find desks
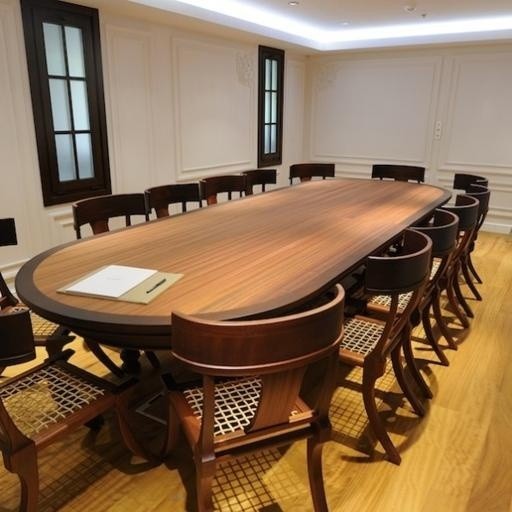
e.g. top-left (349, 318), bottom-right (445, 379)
top-left (14, 177), bottom-right (453, 449)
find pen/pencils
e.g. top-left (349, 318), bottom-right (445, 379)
top-left (147, 279), bottom-right (165, 293)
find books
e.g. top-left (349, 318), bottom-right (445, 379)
top-left (64, 265), bottom-right (158, 299)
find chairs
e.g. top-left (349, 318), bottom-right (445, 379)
top-left (73, 193), bottom-right (161, 368)
top-left (371, 163), bottom-right (427, 184)
top-left (3, 349), bottom-right (161, 510)
top-left (339, 228), bottom-right (425, 465)
top-left (3, 216), bottom-right (125, 380)
top-left (200, 176), bottom-right (252, 206)
top-left (290, 164), bottom-right (335, 190)
top-left (242, 169), bottom-right (277, 196)
top-left (142, 183), bottom-right (204, 223)
top-left (413, 174), bottom-right (490, 366)
top-left (161, 283), bottom-right (344, 512)
